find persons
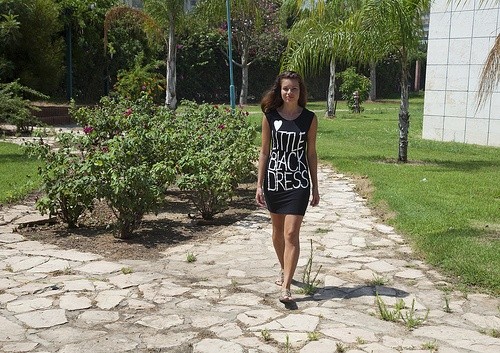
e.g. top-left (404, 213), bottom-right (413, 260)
top-left (255, 70), bottom-right (320, 304)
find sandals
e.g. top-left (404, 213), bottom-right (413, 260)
top-left (279, 288), bottom-right (293, 303)
top-left (276, 269), bottom-right (284, 286)
